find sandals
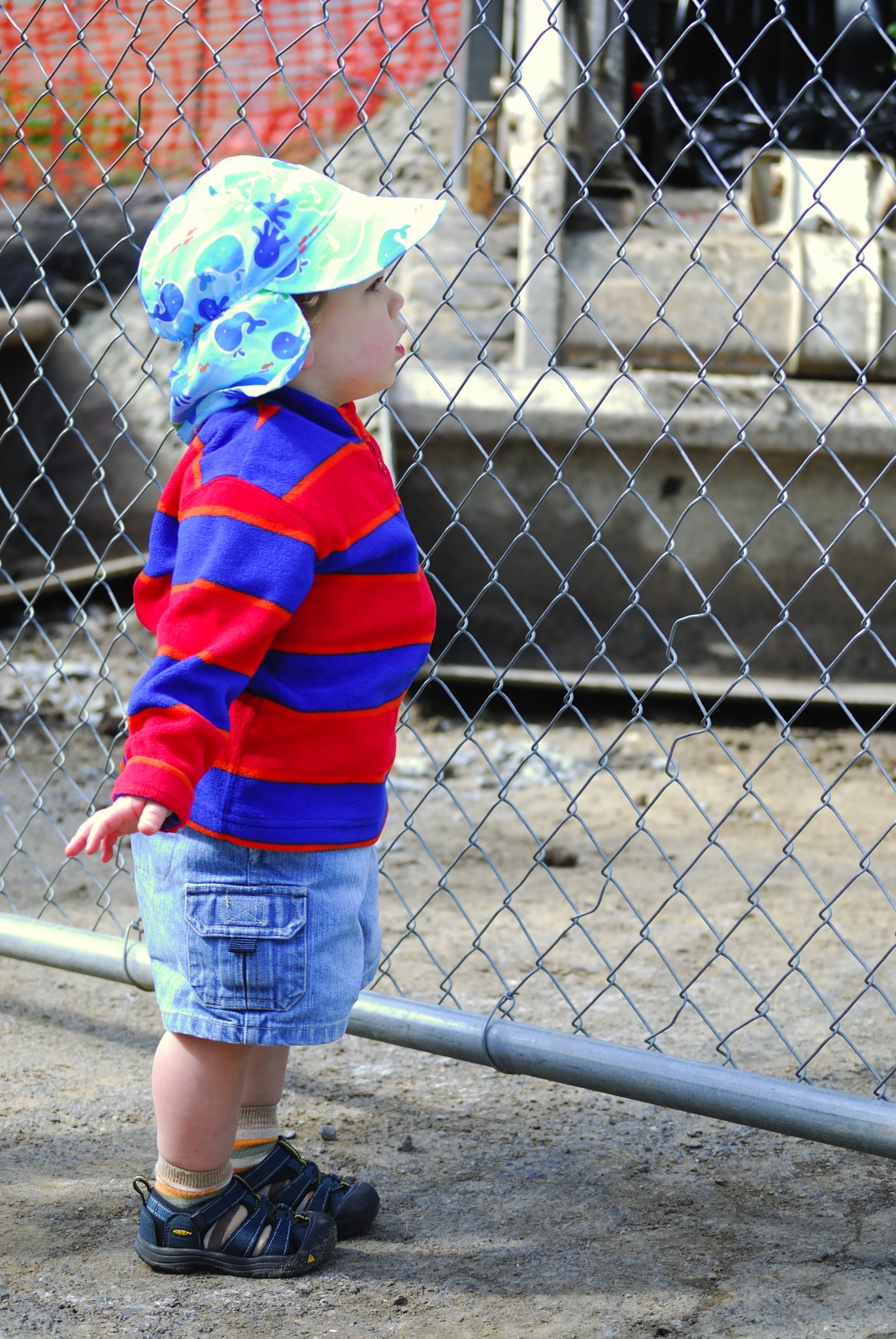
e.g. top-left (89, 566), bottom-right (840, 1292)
top-left (235, 1135), bottom-right (380, 1238)
top-left (132, 1172), bottom-right (337, 1279)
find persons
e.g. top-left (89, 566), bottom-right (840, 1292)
top-left (62, 156), bottom-right (438, 1278)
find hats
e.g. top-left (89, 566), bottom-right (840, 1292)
top-left (138, 155), bottom-right (447, 445)
